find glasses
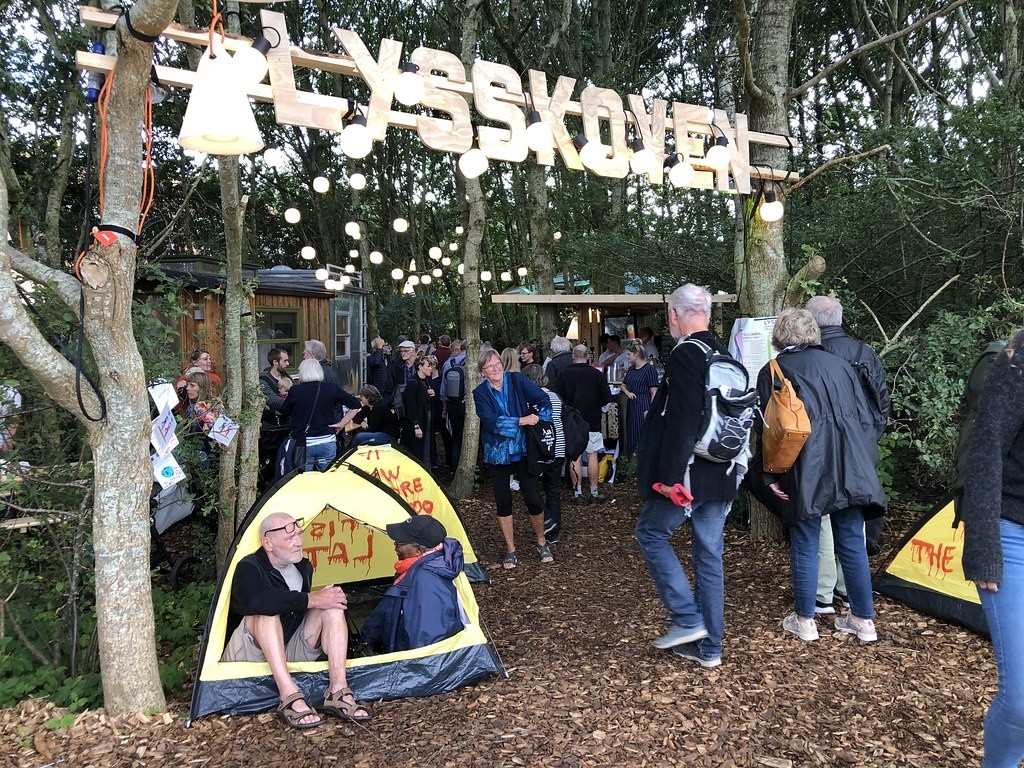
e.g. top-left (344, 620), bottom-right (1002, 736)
top-left (264, 518), bottom-right (305, 537)
top-left (481, 362), bottom-right (502, 370)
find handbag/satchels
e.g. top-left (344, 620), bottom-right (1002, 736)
top-left (560, 403), bottom-right (590, 491)
top-left (526, 404), bottom-right (556, 460)
top-left (316, 610), bottom-right (380, 662)
top-left (274, 425), bottom-right (310, 483)
top-left (762, 358), bottom-right (812, 473)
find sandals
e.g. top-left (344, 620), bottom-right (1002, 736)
top-left (322, 688), bottom-right (372, 720)
top-left (277, 693), bottom-right (324, 729)
top-left (502, 551), bottom-right (517, 569)
top-left (536, 542), bottom-right (554, 563)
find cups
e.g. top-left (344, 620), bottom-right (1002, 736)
top-left (627, 323), bottom-right (635, 339)
top-left (607, 362), bottom-right (618, 382)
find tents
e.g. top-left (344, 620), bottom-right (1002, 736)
top-left (323, 431), bottom-right (492, 586)
top-left (874, 493), bottom-right (992, 639)
top-left (187, 458), bottom-right (510, 729)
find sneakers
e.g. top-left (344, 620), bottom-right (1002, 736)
top-left (654, 625), bottom-right (709, 649)
top-left (782, 612), bottom-right (819, 641)
top-left (589, 494), bottom-right (605, 505)
top-left (834, 609), bottom-right (877, 641)
top-left (574, 494), bottom-right (589, 505)
top-left (672, 641), bottom-right (722, 667)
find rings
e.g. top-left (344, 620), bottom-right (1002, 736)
top-left (774, 492), bottom-right (775, 495)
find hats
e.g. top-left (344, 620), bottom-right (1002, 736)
top-left (397, 341), bottom-right (415, 349)
top-left (386, 515), bottom-right (447, 549)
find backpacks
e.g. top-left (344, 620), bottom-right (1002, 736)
top-left (670, 338), bottom-right (769, 463)
top-left (950, 337), bottom-right (1009, 528)
top-left (444, 357), bottom-right (465, 401)
top-left (826, 343), bottom-right (887, 442)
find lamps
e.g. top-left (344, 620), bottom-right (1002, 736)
top-left (178, 19), bottom-right (264, 155)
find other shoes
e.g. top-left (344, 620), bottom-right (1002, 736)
top-left (815, 599), bottom-right (835, 614)
top-left (833, 586), bottom-right (850, 608)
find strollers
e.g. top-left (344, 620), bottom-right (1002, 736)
top-left (257, 426), bottom-right (291, 493)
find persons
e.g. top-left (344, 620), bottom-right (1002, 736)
top-left (260, 326), bottom-right (663, 490)
top-left (959, 320), bottom-right (1024, 768)
top-left (221, 513), bottom-right (373, 728)
top-left (347, 514), bottom-right (463, 656)
top-left (473, 349), bottom-right (554, 570)
top-left (556, 343), bottom-right (612, 507)
top-left (1, 383), bottom-right (22, 451)
top-left (520, 364), bottom-right (567, 545)
top-left (804, 295), bottom-right (891, 615)
top-left (638, 282), bottom-right (739, 669)
top-left (171, 349), bottom-right (228, 462)
top-left (754, 306), bottom-right (879, 643)
